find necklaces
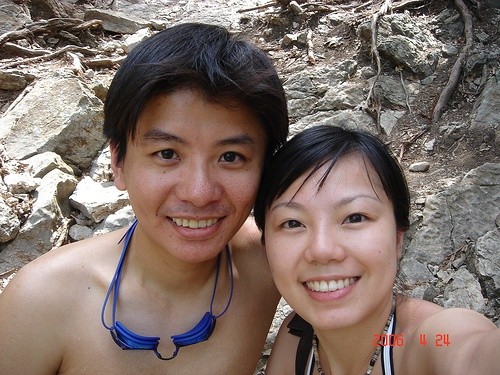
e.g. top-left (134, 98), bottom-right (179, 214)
top-left (311, 293), bottom-right (397, 375)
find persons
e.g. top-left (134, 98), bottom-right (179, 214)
top-left (255, 126), bottom-right (500, 375)
top-left (1, 24), bottom-right (290, 375)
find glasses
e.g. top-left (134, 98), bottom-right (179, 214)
top-left (110, 310), bottom-right (216, 360)
